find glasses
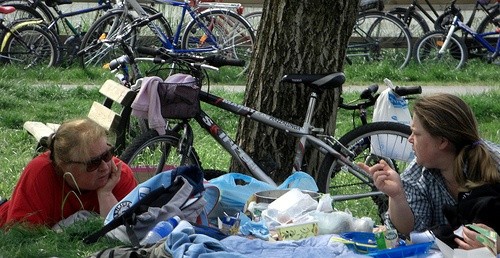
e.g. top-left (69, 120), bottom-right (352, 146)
top-left (68, 143), bottom-right (115, 172)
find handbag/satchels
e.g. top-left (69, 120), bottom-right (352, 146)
top-left (370, 78), bottom-right (417, 163)
top-left (103, 164), bottom-right (220, 245)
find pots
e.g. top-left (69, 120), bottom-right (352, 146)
top-left (254, 189), bottom-right (322, 204)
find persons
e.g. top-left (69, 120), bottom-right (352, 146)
top-left (0, 116), bottom-right (138, 234)
top-left (357, 92), bottom-right (500, 254)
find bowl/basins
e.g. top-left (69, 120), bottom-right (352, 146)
top-left (275, 220), bottom-right (319, 242)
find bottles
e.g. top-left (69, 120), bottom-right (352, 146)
top-left (383, 212), bottom-right (398, 249)
top-left (139, 215), bottom-right (182, 246)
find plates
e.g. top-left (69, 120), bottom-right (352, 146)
top-left (368, 240), bottom-right (435, 258)
top-left (340, 230), bottom-right (377, 253)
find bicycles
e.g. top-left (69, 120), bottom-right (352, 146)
top-left (1, 0), bottom-right (498, 227)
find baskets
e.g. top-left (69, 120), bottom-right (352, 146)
top-left (146, 68), bottom-right (201, 120)
